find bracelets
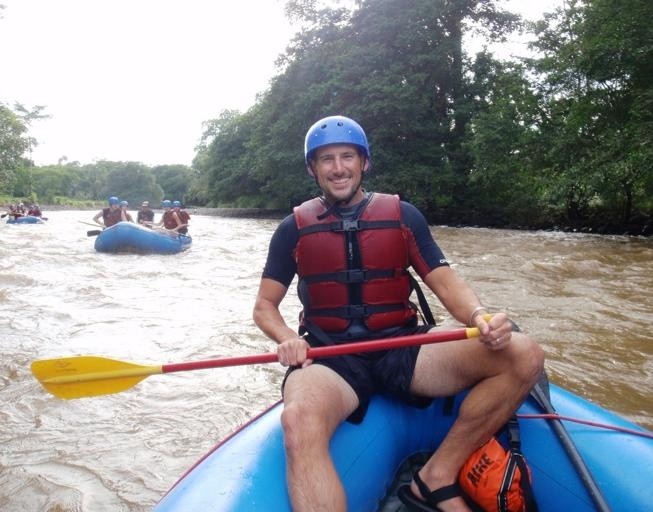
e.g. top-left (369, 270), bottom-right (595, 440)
top-left (467, 304), bottom-right (489, 326)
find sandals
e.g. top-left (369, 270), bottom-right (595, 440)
top-left (396, 469), bottom-right (466, 512)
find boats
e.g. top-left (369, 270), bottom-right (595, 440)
top-left (148, 376), bottom-right (653, 511)
top-left (5, 216), bottom-right (47, 224)
top-left (93, 221), bottom-right (192, 255)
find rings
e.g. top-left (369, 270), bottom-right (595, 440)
top-left (495, 337), bottom-right (501, 344)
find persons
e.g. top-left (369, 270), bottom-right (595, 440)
top-left (251, 113), bottom-right (547, 510)
top-left (8, 200), bottom-right (42, 219)
top-left (142, 200), bottom-right (182, 237)
top-left (136, 199), bottom-right (154, 230)
top-left (117, 200), bottom-right (135, 224)
top-left (91, 196), bottom-right (127, 229)
top-left (160, 201), bottom-right (191, 236)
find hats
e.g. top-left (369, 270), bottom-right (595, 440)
top-left (141, 201), bottom-right (149, 207)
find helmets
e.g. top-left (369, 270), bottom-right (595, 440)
top-left (108, 196), bottom-right (120, 207)
top-left (120, 201), bottom-right (129, 207)
top-left (9, 202), bottom-right (39, 212)
top-left (173, 201), bottom-right (182, 207)
top-left (161, 199), bottom-right (173, 208)
top-left (302, 112), bottom-right (371, 176)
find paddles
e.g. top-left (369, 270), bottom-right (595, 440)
top-left (87, 231), bottom-right (100, 236)
top-left (31, 314), bottom-right (492, 400)
top-left (1, 208), bottom-right (16, 218)
